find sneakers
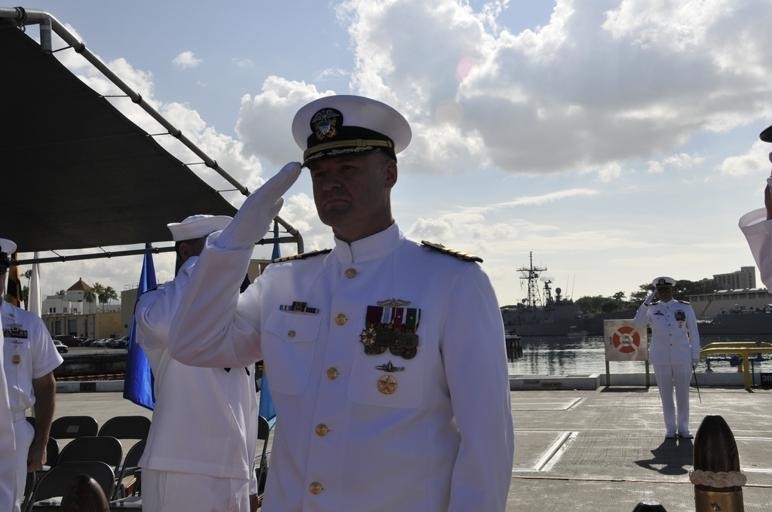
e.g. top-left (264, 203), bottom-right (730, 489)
top-left (664, 433), bottom-right (693, 441)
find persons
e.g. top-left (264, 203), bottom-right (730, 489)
top-left (633, 276), bottom-right (701, 439)
top-left (166, 95), bottom-right (515, 512)
top-left (133, 215), bottom-right (261, 512)
top-left (0, 238), bottom-right (57, 512)
top-left (739, 124), bottom-right (772, 293)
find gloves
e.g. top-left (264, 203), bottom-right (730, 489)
top-left (214, 161), bottom-right (302, 249)
top-left (644, 292), bottom-right (655, 306)
top-left (692, 359), bottom-right (701, 370)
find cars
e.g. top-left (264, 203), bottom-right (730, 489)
top-left (54, 335), bottom-right (129, 354)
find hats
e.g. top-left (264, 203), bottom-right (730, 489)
top-left (650, 277), bottom-right (678, 290)
top-left (0, 236), bottom-right (18, 274)
top-left (290, 94), bottom-right (414, 170)
top-left (167, 212), bottom-right (234, 243)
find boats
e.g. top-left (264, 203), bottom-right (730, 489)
top-left (696, 307), bottom-right (770, 337)
top-left (501, 247), bottom-right (580, 332)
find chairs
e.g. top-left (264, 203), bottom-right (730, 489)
top-left (21, 409), bottom-right (273, 512)
